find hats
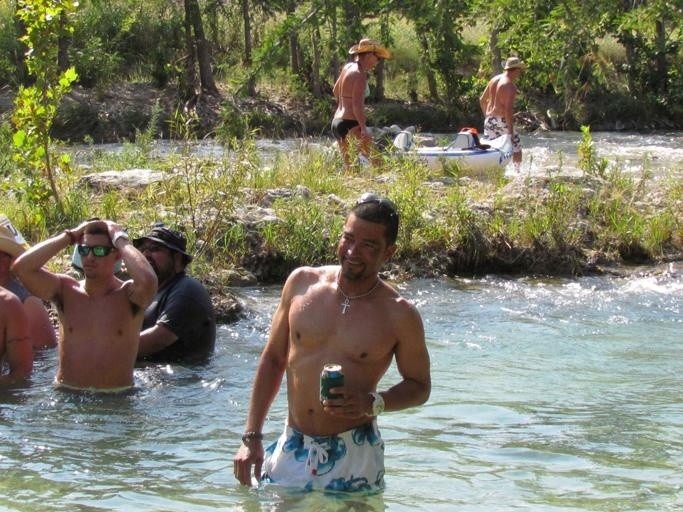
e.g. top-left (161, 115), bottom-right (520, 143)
top-left (500, 56), bottom-right (528, 70)
top-left (132, 226), bottom-right (192, 267)
top-left (348, 39), bottom-right (391, 59)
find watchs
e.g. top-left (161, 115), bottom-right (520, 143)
top-left (364, 390), bottom-right (384, 418)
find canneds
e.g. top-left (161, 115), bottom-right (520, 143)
top-left (319, 363), bottom-right (345, 408)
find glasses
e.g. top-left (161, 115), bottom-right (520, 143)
top-left (76, 244), bottom-right (118, 258)
top-left (355, 191), bottom-right (399, 218)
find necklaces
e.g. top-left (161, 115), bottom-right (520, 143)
top-left (336, 269), bottom-right (381, 315)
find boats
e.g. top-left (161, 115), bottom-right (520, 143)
top-left (364, 133), bottom-right (512, 175)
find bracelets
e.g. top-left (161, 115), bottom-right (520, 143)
top-left (240, 431), bottom-right (266, 442)
top-left (64, 228), bottom-right (75, 247)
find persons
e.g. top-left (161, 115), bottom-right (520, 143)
top-left (0, 286), bottom-right (34, 386)
top-left (12, 216), bottom-right (159, 392)
top-left (118, 225), bottom-right (216, 362)
top-left (330, 38), bottom-right (392, 172)
top-left (478, 56), bottom-right (527, 175)
top-left (0, 214), bottom-right (58, 349)
top-left (232, 199), bottom-right (432, 495)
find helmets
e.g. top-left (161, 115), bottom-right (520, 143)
top-left (0, 213), bottom-right (30, 259)
